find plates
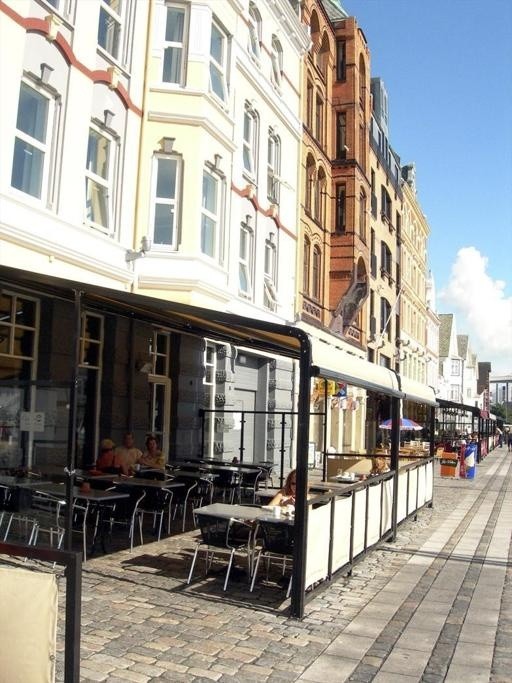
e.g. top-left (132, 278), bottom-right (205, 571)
top-left (262, 506), bottom-right (285, 510)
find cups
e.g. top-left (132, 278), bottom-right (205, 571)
top-left (273, 506), bottom-right (279, 516)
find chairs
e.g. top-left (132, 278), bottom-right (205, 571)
top-left (144, 486), bottom-right (174, 542)
top-left (0, 482), bottom-right (16, 542)
top-left (249, 519), bottom-right (296, 599)
top-left (91, 484), bottom-right (145, 552)
top-left (212, 468), bottom-right (243, 505)
top-left (175, 480), bottom-right (198, 533)
top-left (244, 471), bottom-right (262, 504)
top-left (14, 485), bottom-right (67, 550)
top-left (187, 512), bottom-right (261, 592)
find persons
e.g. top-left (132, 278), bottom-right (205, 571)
top-left (95, 437), bottom-right (124, 475)
top-left (267, 469), bottom-right (296, 509)
top-left (507, 432), bottom-right (512, 452)
top-left (499, 432), bottom-right (503, 448)
top-left (115, 432), bottom-right (140, 475)
top-left (139, 435), bottom-right (166, 471)
top-left (367, 453), bottom-right (390, 481)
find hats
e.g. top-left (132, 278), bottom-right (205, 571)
top-left (101, 440), bottom-right (116, 449)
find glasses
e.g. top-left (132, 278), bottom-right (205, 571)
top-left (290, 482), bottom-right (297, 485)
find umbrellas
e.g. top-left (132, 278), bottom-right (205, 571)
top-left (379, 417), bottom-right (423, 432)
